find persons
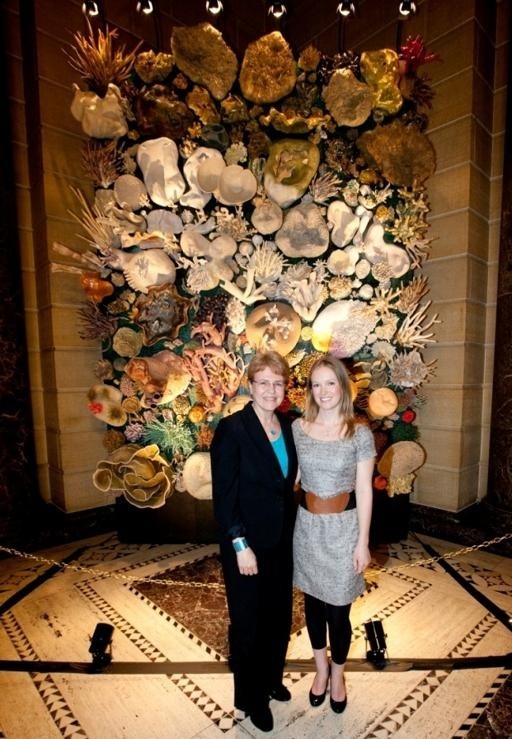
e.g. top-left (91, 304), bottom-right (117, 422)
top-left (207, 350), bottom-right (307, 733)
top-left (289, 355), bottom-right (379, 714)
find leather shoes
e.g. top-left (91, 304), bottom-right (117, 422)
top-left (330, 693), bottom-right (346, 714)
top-left (248, 702), bottom-right (273, 731)
top-left (268, 683), bottom-right (291, 701)
top-left (309, 688), bottom-right (326, 706)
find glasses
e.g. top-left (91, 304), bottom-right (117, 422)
top-left (251, 379), bottom-right (285, 388)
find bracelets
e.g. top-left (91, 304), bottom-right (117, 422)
top-left (232, 540), bottom-right (252, 556)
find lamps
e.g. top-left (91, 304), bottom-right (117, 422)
top-left (364, 620), bottom-right (389, 671)
top-left (87, 622), bottom-right (115, 672)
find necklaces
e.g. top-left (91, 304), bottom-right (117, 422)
top-left (252, 403), bottom-right (277, 438)
